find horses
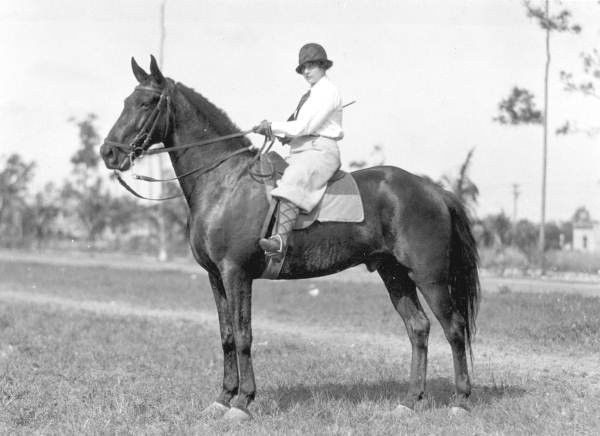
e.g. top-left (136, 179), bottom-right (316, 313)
top-left (99, 53), bottom-right (481, 423)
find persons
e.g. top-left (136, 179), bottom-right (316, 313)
top-left (253, 42), bottom-right (353, 263)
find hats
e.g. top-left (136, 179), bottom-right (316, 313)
top-left (295, 43), bottom-right (333, 73)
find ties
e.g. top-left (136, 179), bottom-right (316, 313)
top-left (289, 89), bottom-right (311, 120)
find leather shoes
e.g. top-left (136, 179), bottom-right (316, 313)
top-left (258, 239), bottom-right (287, 263)
